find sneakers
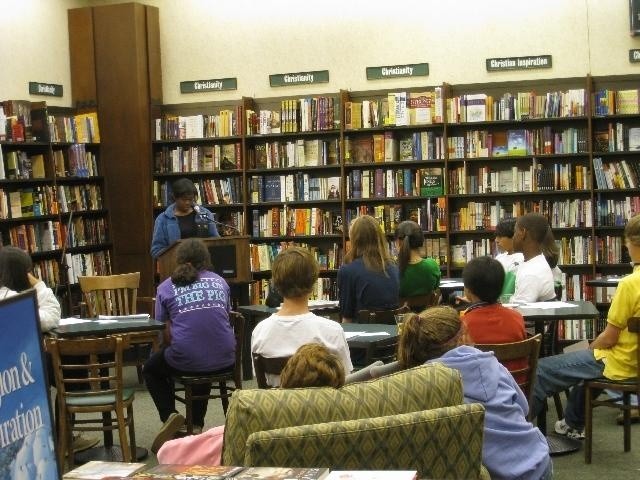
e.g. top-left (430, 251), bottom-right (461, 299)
top-left (65, 431), bottom-right (101, 456)
top-left (616, 409), bottom-right (640, 425)
top-left (555, 417), bottom-right (586, 440)
top-left (177, 422), bottom-right (202, 435)
top-left (151, 413), bottom-right (185, 454)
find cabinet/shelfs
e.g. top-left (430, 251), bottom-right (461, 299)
top-left (0, 97), bottom-right (120, 318)
top-left (146, 73), bottom-right (640, 354)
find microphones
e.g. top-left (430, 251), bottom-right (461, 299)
top-left (194, 205), bottom-right (207, 221)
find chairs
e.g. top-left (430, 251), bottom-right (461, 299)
top-left (45, 334), bottom-right (136, 474)
top-left (167, 311), bottom-right (246, 436)
top-left (77, 272), bottom-right (151, 384)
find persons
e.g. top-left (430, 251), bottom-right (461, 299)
top-left (457, 257), bottom-right (531, 405)
top-left (157, 343), bottom-right (346, 465)
top-left (1, 245), bottom-right (100, 457)
top-left (604, 387), bottom-right (640, 424)
top-left (398, 308), bottom-right (554, 480)
top-left (524, 217), bottom-right (640, 441)
top-left (250, 248), bottom-right (354, 389)
top-left (142, 239), bottom-right (236, 454)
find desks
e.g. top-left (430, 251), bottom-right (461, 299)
top-left (42, 315), bottom-right (167, 465)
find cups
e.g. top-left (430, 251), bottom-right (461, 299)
top-left (500, 293), bottom-right (513, 311)
top-left (394, 313), bottom-right (406, 335)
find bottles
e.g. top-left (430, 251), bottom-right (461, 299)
top-left (79, 302), bottom-right (86, 319)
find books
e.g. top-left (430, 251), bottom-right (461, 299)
top-left (64, 461), bottom-right (329, 480)
top-left (0, 88), bottom-right (640, 338)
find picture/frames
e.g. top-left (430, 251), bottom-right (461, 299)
top-left (0, 289), bottom-right (61, 480)
